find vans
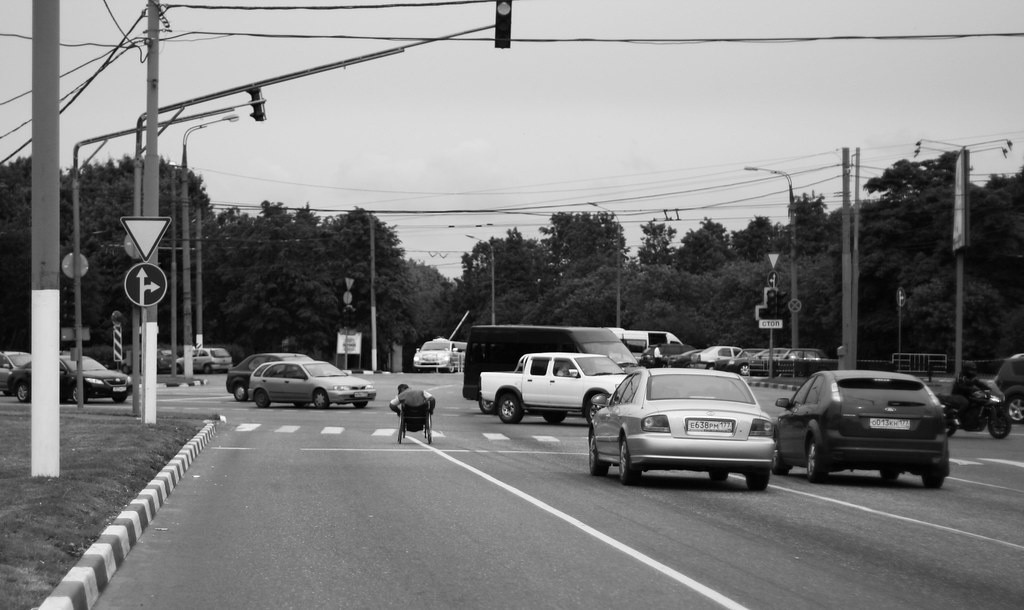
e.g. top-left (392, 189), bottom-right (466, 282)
top-left (612, 327), bottom-right (681, 364)
top-left (461, 325), bottom-right (639, 412)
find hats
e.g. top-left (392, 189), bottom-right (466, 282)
top-left (397, 384), bottom-right (408, 394)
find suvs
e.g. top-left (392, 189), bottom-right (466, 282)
top-left (412, 340), bottom-right (467, 373)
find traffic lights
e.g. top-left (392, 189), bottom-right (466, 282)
top-left (495, 1), bottom-right (513, 49)
top-left (247, 89), bottom-right (264, 121)
top-left (761, 290), bottom-right (790, 321)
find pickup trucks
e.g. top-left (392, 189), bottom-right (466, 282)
top-left (479, 352), bottom-right (631, 425)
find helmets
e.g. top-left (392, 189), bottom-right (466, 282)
top-left (962, 362), bottom-right (977, 377)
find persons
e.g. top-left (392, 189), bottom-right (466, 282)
top-left (556, 362), bottom-right (570, 377)
top-left (390, 384), bottom-right (436, 417)
top-left (654, 344), bottom-right (662, 367)
top-left (951, 361), bottom-right (993, 426)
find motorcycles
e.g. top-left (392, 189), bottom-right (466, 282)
top-left (939, 376), bottom-right (1011, 440)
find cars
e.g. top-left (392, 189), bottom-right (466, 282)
top-left (640, 344), bottom-right (830, 375)
top-left (586, 366), bottom-right (774, 490)
top-left (995, 355), bottom-right (1024, 421)
top-left (0, 350), bottom-right (31, 393)
top-left (7, 357), bottom-right (133, 405)
top-left (772, 374), bottom-right (951, 490)
top-left (226, 352), bottom-right (378, 408)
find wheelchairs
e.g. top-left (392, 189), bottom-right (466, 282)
top-left (398, 401), bottom-right (432, 444)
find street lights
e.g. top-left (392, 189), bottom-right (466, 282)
top-left (181, 115), bottom-right (240, 379)
top-left (745, 168), bottom-right (799, 348)
top-left (465, 235), bottom-right (495, 326)
top-left (588, 203), bottom-right (620, 327)
